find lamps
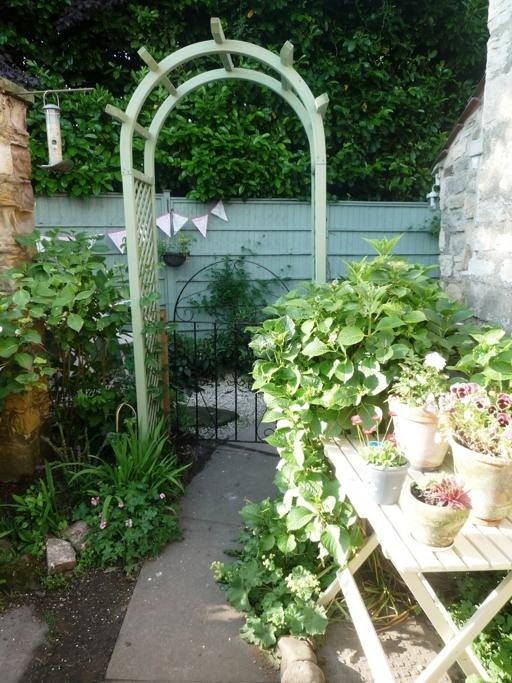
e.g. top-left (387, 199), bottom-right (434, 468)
top-left (425, 183), bottom-right (439, 210)
top-left (158, 231), bottom-right (191, 267)
top-left (43, 88), bottom-right (63, 166)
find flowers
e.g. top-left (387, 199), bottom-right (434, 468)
top-left (351, 407), bottom-right (409, 466)
top-left (438, 382), bottom-right (512, 460)
top-left (423, 472), bottom-right (472, 510)
top-left (385, 349), bottom-right (449, 407)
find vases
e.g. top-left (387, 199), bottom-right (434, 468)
top-left (447, 434), bottom-right (511, 523)
top-left (388, 398), bottom-right (451, 470)
top-left (409, 482), bottom-right (473, 551)
top-left (360, 456), bottom-right (411, 506)
